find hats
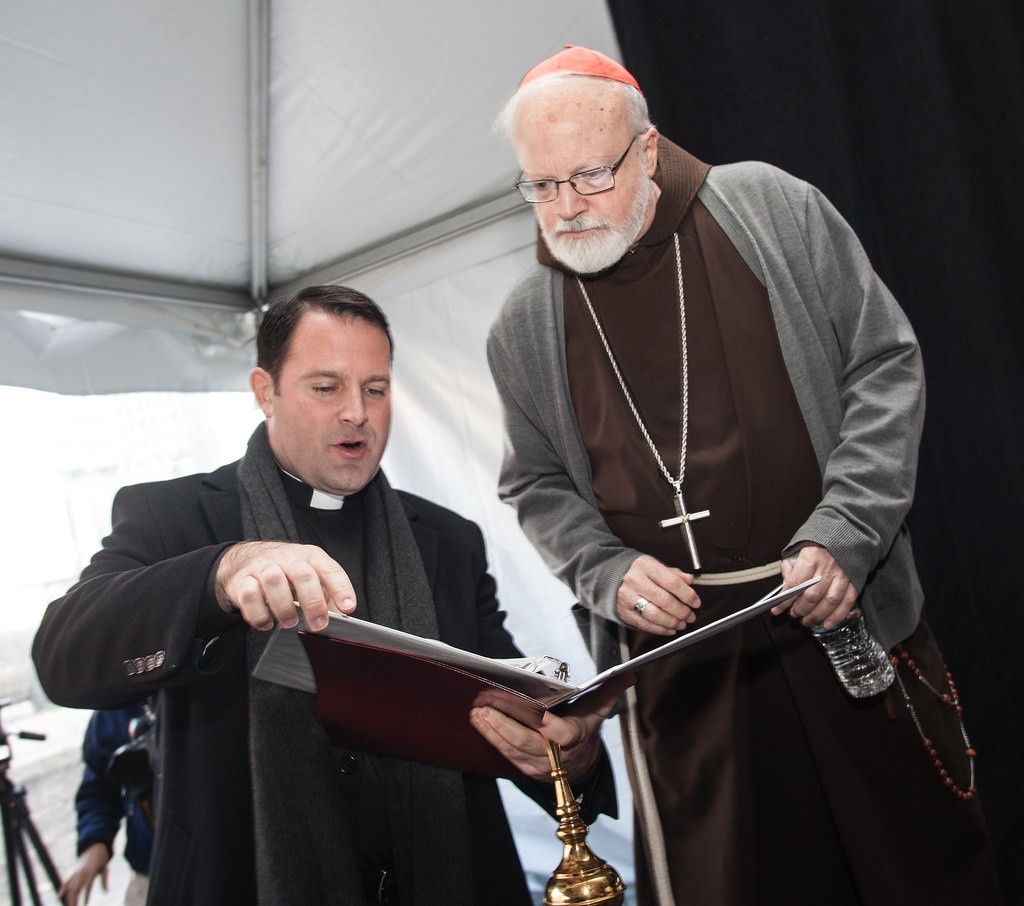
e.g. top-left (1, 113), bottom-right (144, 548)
top-left (517, 44), bottom-right (641, 90)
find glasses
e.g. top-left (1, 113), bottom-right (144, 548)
top-left (513, 131), bottom-right (637, 204)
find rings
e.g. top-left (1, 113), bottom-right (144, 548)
top-left (634, 598), bottom-right (650, 614)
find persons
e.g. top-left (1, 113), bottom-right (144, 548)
top-left (480, 46), bottom-right (1002, 906)
top-left (56, 702), bottom-right (161, 906)
top-left (34, 280), bottom-right (624, 906)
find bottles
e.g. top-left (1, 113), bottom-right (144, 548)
top-left (789, 554), bottom-right (896, 699)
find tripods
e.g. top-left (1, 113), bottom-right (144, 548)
top-left (0, 732), bottom-right (71, 906)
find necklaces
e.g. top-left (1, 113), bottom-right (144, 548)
top-left (577, 232), bottom-right (711, 571)
